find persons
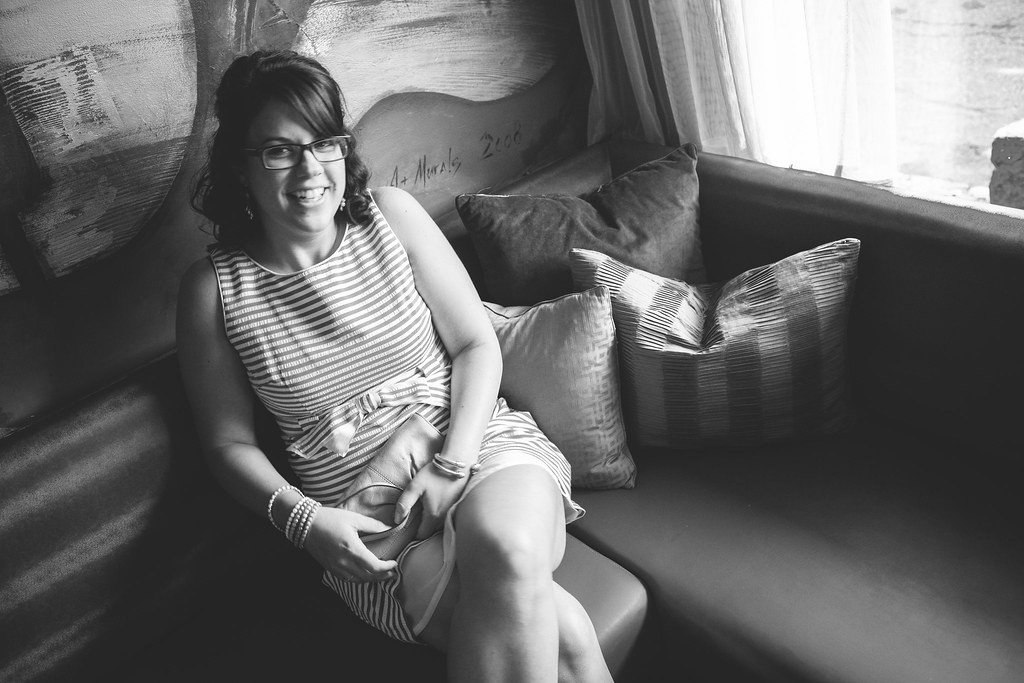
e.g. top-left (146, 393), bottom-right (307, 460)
top-left (176, 49), bottom-right (614, 683)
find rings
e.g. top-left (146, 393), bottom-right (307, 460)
top-left (344, 575), bottom-right (354, 584)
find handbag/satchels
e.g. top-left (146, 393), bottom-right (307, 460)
top-left (333, 412), bottom-right (444, 561)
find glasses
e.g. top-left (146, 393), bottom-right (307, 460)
top-left (242, 135), bottom-right (351, 169)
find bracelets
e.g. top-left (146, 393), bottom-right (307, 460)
top-left (267, 485), bottom-right (305, 532)
top-left (432, 452), bottom-right (483, 479)
top-left (285, 495), bottom-right (323, 550)
